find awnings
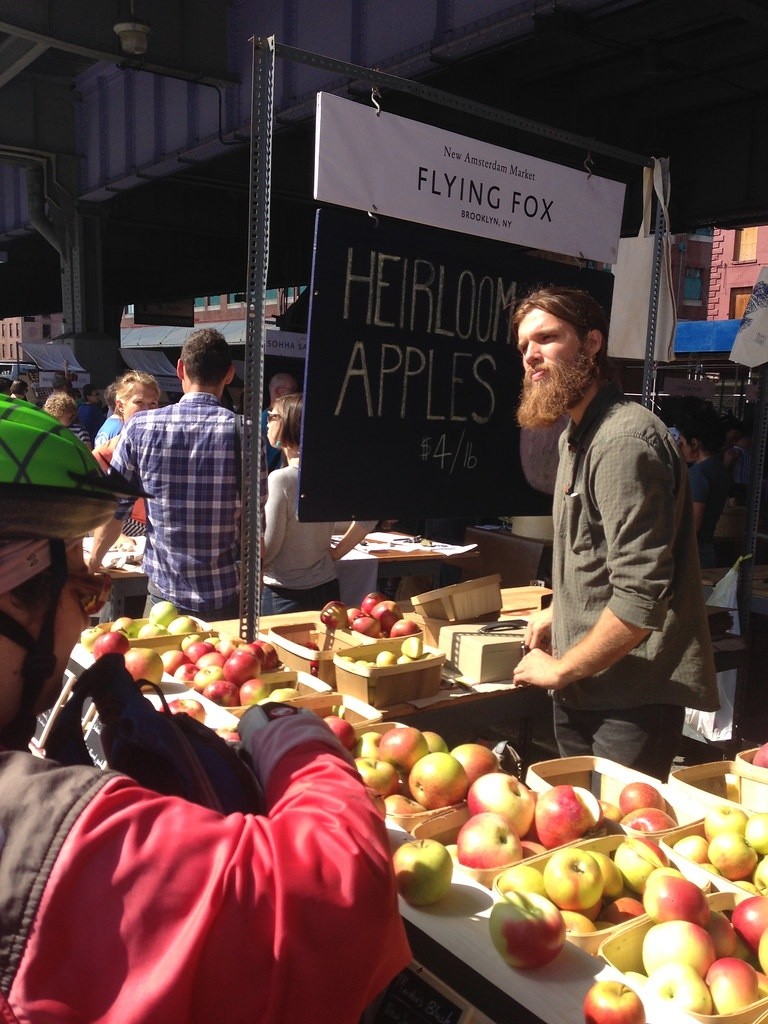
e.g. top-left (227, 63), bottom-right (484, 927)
top-left (15, 342), bottom-right (86, 382)
top-left (117, 348), bottom-right (179, 378)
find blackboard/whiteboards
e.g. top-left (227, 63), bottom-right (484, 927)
top-left (295, 206), bottom-right (621, 528)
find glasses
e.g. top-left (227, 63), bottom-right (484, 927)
top-left (41, 569), bottom-right (114, 615)
top-left (266, 410), bottom-right (281, 423)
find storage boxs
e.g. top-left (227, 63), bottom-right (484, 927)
top-left (189, 668), bottom-right (767, 1023)
top-left (268, 618), bottom-right (447, 710)
top-left (95, 613), bottom-right (219, 648)
top-left (410, 571), bottom-right (503, 649)
top-left (439, 620), bottom-right (530, 684)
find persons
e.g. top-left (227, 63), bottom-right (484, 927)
top-left (0, 391), bottom-right (412, 1024)
top-left (661, 397), bottom-right (755, 571)
top-left (0, 330), bottom-right (400, 622)
top-left (511, 285), bottom-right (723, 784)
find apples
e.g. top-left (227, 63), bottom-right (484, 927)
top-left (323, 714), bottom-right (768, 1024)
top-left (81, 590), bottom-right (436, 743)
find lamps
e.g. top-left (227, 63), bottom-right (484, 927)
top-left (112, 0), bottom-right (152, 55)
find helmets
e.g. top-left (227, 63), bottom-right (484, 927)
top-left (0, 393), bottom-right (155, 543)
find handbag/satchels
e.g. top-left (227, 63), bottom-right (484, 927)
top-left (43, 652), bottom-right (265, 820)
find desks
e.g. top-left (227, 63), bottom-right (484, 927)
top-left (81, 529), bottom-right (481, 624)
top-left (700, 565), bottom-right (768, 617)
top-left (384, 813), bottom-right (698, 1024)
top-left (78, 583), bottom-right (754, 783)
